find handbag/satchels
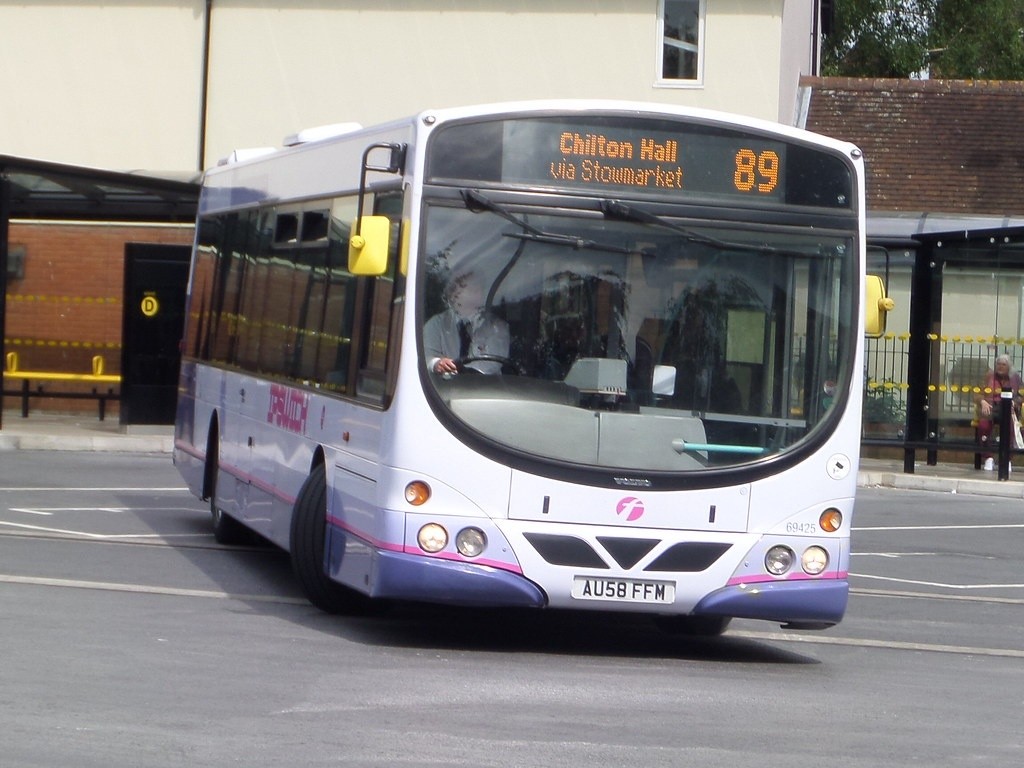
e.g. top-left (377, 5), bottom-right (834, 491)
top-left (1010, 411), bottom-right (1024, 449)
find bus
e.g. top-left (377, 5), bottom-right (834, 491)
top-left (171, 97), bottom-right (896, 638)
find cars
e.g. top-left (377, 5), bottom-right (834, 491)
top-left (948, 355), bottom-right (991, 401)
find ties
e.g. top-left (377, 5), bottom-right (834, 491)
top-left (458, 319), bottom-right (473, 359)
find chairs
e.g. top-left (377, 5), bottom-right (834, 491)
top-left (586, 332), bottom-right (657, 408)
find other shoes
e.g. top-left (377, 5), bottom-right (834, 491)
top-left (1009, 462), bottom-right (1012, 472)
top-left (984, 457), bottom-right (995, 471)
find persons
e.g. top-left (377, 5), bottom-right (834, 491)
top-left (973, 353), bottom-right (1024, 473)
top-left (420, 266), bottom-right (516, 386)
